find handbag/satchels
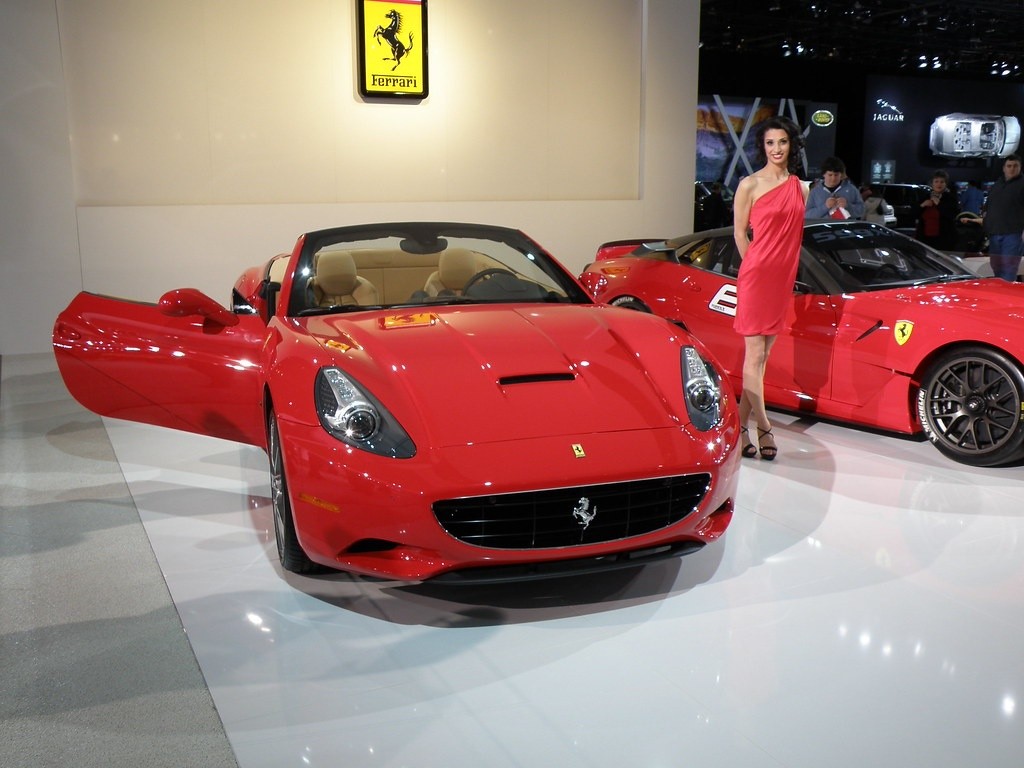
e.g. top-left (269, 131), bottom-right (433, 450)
top-left (876, 199), bottom-right (883, 215)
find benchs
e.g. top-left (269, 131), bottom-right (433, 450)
top-left (315, 249), bottom-right (441, 305)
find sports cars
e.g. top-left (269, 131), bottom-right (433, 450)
top-left (52, 220), bottom-right (744, 589)
top-left (579, 220), bottom-right (1024, 471)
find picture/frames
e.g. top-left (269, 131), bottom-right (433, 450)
top-left (358, 0), bottom-right (430, 99)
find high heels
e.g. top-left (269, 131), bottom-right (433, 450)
top-left (741, 426), bottom-right (757, 457)
top-left (757, 427), bottom-right (777, 460)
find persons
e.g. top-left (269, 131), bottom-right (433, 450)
top-left (911, 169), bottom-right (952, 246)
top-left (806, 157), bottom-right (893, 263)
top-left (960, 155), bottom-right (1024, 281)
top-left (961, 180), bottom-right (984, 215)
top-left (734, 116), bottom-right (809, 459)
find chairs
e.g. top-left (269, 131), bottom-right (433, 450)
top-left (423, 248), bottom-right (489, 301)
top-left (314, 251), bottom-right (378, 306)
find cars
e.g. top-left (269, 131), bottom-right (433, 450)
top-left (694, 180), bottom-right (736, 218)
top-left (927, 113), bottom-right (1022, 162)
top-left (860, 183), bottom-right (932, 225)
